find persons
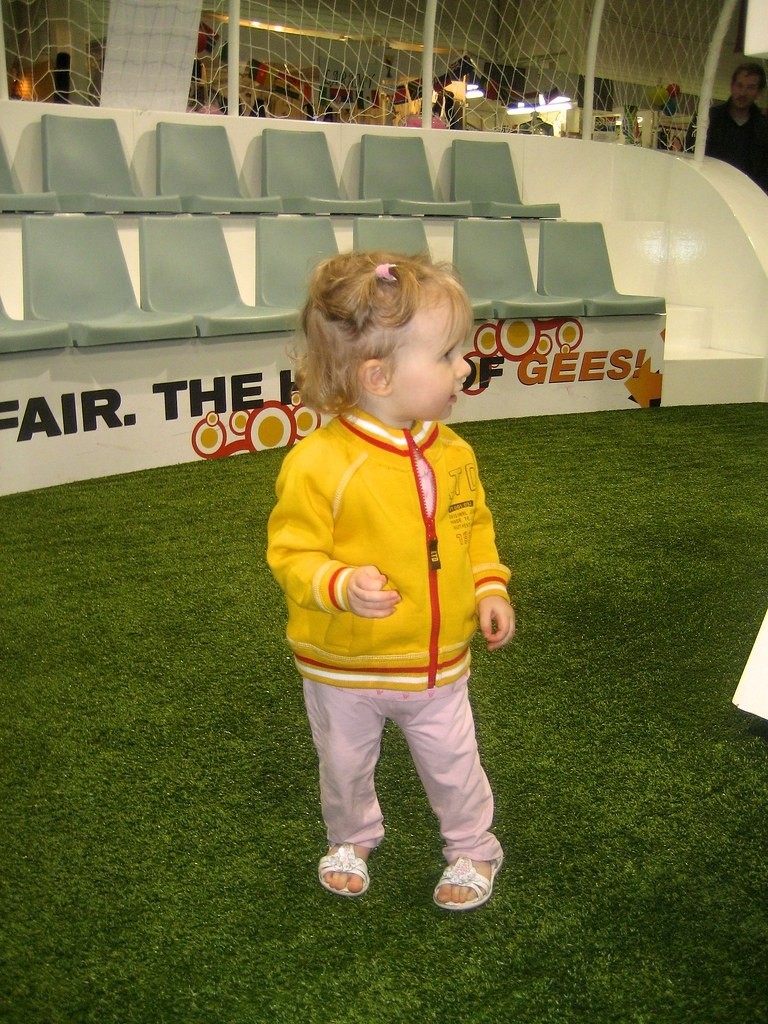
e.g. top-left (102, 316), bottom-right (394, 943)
top-left (683, 63), bottom-right (768, 198)
top-left (267, 250), bottom-right (514, 910)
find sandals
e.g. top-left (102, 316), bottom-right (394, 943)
top-left (318, 837), bottom-right (373, 896)
top-left (433, 855), bottom-right (502, 911)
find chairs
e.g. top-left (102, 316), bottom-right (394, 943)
top-left (260, 127), bottom-right (384, 216)
top-left (41, 114), bottom-right (182, 215)
top-left (139, 215), bottom-right (300, 337)
top-left (0, 137), bottom-right (59, 214)
top-left (0, 295), bottom-right (74, 353)
top-left (450, 139), bottom-right (561, 220)
top-left (352, 217), bottom-right (495, 319)
top-left (451, 218), bottom-right (586, 319)
top-left (156, 121), bottom-right (284, 214)
top-left (536, 220), bottom-right (666, 318)
top-left (254, 215), bottom-right (339, 330)
top-left (358, 133), bottom-right (473, 218)
top-left (21, 215), bottom-right (197, 348)
